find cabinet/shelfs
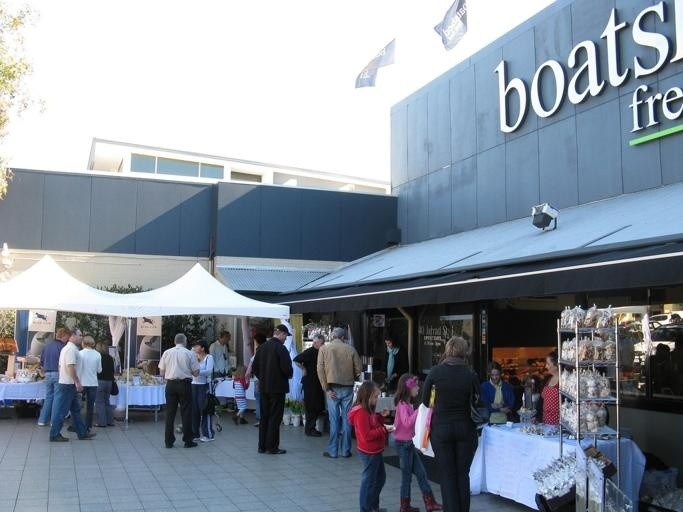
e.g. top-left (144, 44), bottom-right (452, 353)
top-left (556, 316), bottom-right (619, 490)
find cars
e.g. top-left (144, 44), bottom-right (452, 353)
top-left (622, 309), bottom-right (683, 362)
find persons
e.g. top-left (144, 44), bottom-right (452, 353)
top-left (231, 365), bottom-right (249, 425)
top-left (158, 333), bottom-right (216, 449)
top-left (244, 334), bottom-right (267, 427)
top-left (317, 329), bottom-right (364, 458)
top-left (373, 333), bottom-right (408, 388)
top-left (208, 331), bottom-right (231, 377)
top-left (541, 349), bottom-right (558, 425)
top-left (37, 327), bottom-right (117, 442)
top-left (294, 335), bottom-right (325, 437)
top-left (391, 373), bottom-right (444, 511)
top-left (645, 340), bottom-right (671, 388)
top-left (350, 381), bottom-right (398, 511)
top-left (252, 324), bottom-right (293, 454)
top-left (421, 335), bottom-right (479, 511)
top-left (478, 362), bottom-right (517, 423)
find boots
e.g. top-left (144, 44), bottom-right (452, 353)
top-left (423, 493), bottom-right (444, 512)
top-left (400, 495), bottom-right (420, 512)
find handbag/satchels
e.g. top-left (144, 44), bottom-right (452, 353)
top-left (468, 373), bottom-right (490, 424)
top-left (110, 382), bottom-right (119, 395)
top-left (200, 392), bottom-right (221, 416)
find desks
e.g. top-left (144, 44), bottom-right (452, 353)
top-left (468, 421), bottom-right (646, 512)
top-left (213, 377), bottom-right (256, 408)
top-left (0, 377), bottom-right (48, 419)
top-left (108, 378), bottom-right (166, 424)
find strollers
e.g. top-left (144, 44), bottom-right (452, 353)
top-left (175, 395), bottom-right (225, 434)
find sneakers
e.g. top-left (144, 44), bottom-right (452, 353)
top-left (232, 415), bottom-right (248, 425)
top-left (184, 436), bottom-right (214, 447)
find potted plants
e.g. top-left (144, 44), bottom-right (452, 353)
top-left (282, 397), bottom-right (324, 431)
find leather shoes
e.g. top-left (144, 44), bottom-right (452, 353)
top-left (323, 452), bottom-right (337, 458)
top-left (343, 453), bottom-right (352, 457)
top-left (305, 429), bottom-right (321, 436)
top-left (53, 434), bottom-right (69, 441)
top-left (259, 447), bottom-right (286, 454)
top-left (78, 431), bottom-right (97, 439)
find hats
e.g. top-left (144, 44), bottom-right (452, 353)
top-left (274, 324), bottom-right (292, 336)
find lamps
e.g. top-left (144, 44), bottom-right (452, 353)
top-left (529, 202), bottom-right (559, 232)
